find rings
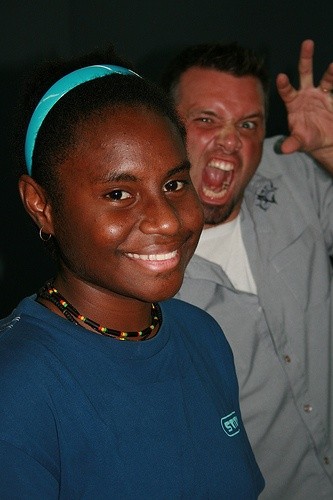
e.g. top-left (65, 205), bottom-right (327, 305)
top-left (320, 86), bottom-right (329, 92)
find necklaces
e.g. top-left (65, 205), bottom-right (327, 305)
top-left (38, 280), bottom-right (160, 342)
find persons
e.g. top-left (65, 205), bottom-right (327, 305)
top-left (159, 39), bottom-right (333, 500)
top-left (0, 62), bottom-right (267, 499)
